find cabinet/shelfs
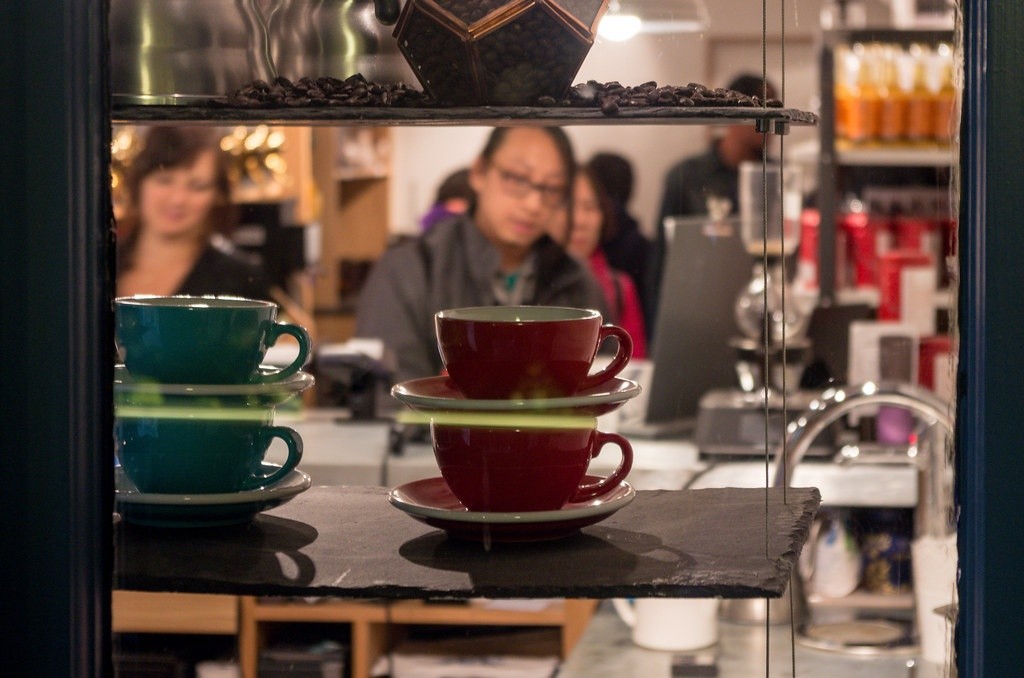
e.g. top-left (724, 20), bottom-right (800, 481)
top-left (816, 22), bottom-right (964, 443)
top-left (298, 124), bottom-right (393, 403)
top-left (108, 106), bottom-right (821, 601)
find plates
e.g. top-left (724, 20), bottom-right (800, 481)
top-left (112, 363), bottom-right (314, 409)
top-left (388, 474), bottom-right (636, 543)
top-left (112, 463), bottom-right (311, 520)
top-left (391, 370), bottom-right (643, 415)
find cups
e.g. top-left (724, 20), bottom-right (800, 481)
top-left (113, 292), bottom-right (312, 386)
top-left (429, 415), bottom-right (633, 515)
top-left (435, 305), bottom-right (633, 399)
top-left (113, 407), bottom-right (304, 496)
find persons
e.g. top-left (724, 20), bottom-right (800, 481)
top-left (429, 74), bottom-right (784, 353)
top-left (114, 126), bottom-right (269, 300)
top-left (355, 124), bottom-right (613, 383)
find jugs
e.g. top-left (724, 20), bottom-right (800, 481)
top-left (611, 460), bottom-right (726, 648)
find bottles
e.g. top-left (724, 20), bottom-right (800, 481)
top-left (792, 191), bottom-right (938, 295)
top-left (829, 42), bottom-right (963, 146)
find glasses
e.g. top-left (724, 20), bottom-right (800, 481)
top-left (488, 158), bottom-right (571, 209)
